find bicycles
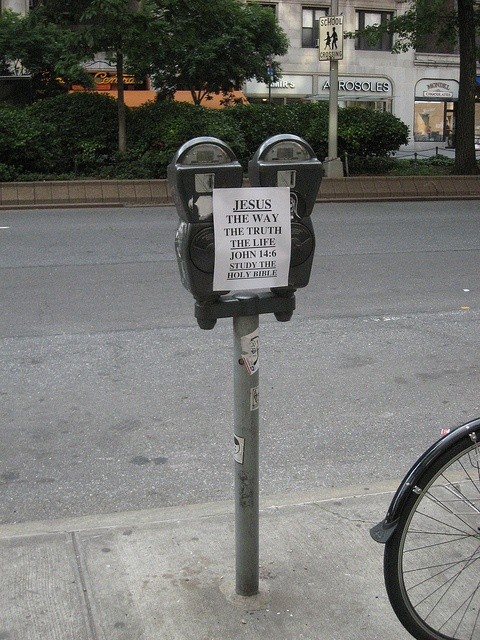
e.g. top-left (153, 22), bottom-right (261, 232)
top-left (369, 419), bottom-right (480, 639)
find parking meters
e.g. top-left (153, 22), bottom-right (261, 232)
top-left (167, 133), bottom-right (321, 593)
